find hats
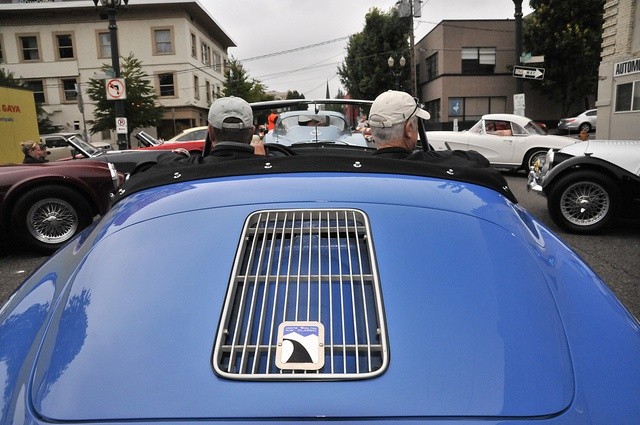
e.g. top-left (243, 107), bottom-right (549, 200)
top-left (208, 96), bottom-right (253, 130)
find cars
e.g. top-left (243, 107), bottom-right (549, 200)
top-left (526, 140), bottom-right (640, 235)
top-left (0, 160), bottom-right (131, 256)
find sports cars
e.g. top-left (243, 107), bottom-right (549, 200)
top-left (557, 108), bottom-right (597, 134)
top-left (0, 97), bottom-right (640, 425)
top-left (263, 110), bottom-right (371, 147)
top-left (40, 126), bottom-right (266, 161)
top-left (425, 113), bottom-right (583, 181)
top-left (68, 135), bottom-right (192, 178)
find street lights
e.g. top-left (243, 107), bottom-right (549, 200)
top-left (388, 56), bottom-right (409, 91)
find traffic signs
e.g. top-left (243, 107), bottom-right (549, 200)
top-left (513, 65), bottom-right (545, 81)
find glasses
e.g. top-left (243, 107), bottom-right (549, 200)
top-left (404, 96), bottom-right (419, 124)
top-left (368, 90), bottom-right (431, 128)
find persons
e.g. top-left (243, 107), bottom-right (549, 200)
top-left (268, 108), bottom-right (278, 130)
top-left (155, 95), bottom-right (267, 168)
top-left (357, 115), bottom-right (368, 132)
top-left (367, 90), bottom-right (491, 169)
top-left (487, 121), bottom-right (512, 135)
top-left (20, 140), bottom-right (81, 163)
